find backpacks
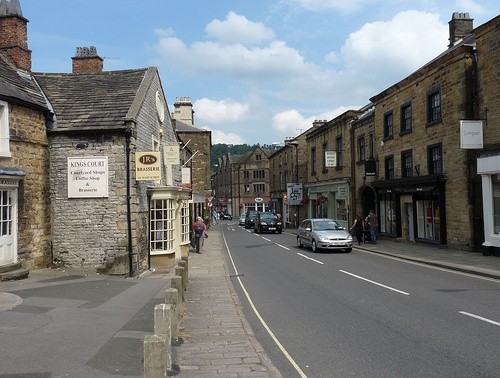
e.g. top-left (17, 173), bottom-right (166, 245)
top-left (195, 222), bottom-right (203, 238)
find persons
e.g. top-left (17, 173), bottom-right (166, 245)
top-left (350, 210), bottom-right (378, 245)
top-left (211, 211), bottom-right (224, 226)
top-left (192, 216), bottom-right (206, 253)
top-left (277, 211), bottom-right (281, 219)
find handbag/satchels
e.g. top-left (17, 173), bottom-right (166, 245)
top-left (190, 237), bottom-right (196, 248)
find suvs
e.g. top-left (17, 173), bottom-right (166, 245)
top-left (245, 210), bottom-right (261, 229)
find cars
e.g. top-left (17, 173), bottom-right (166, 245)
top-left (254, 211), bottom-right (283, 234)
top-left (296, 218), bottom-right (353, 253)
top-left (220, 213), bottom-right (233, 221)
top-left (238, 214), bottom-right (246, 226)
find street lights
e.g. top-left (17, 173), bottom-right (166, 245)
top-left (288, 142), bottom-right (300, 228)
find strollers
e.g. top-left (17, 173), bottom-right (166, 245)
top-left (361, 223), bottom-right (379, 244)
